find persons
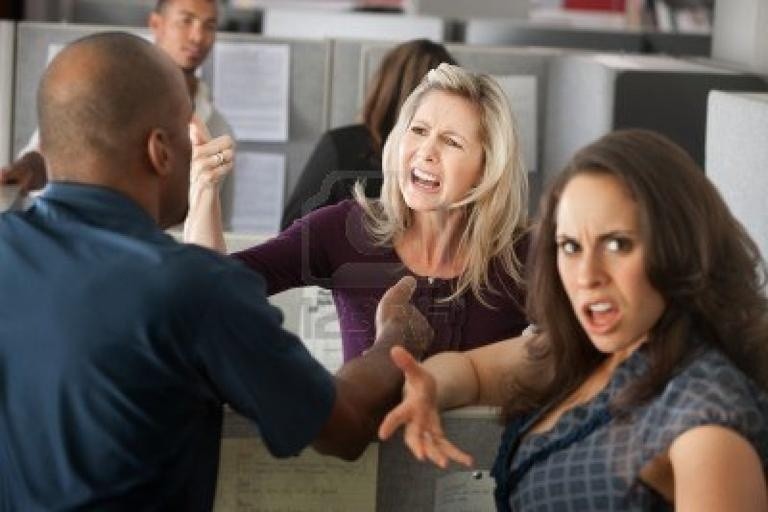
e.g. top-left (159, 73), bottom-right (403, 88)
top-left (379, 131), bottom-right (768, 511)
top-left (1, 0), bottom-right (242, 234)
top-left (189, 61), bottom-right (531, 364)
top-left (281, 39), bottom-right (458, 236)
top-left (0, 31), bottom-right (436, 512)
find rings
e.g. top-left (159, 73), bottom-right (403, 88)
top-left (215, 152), bottom-right (225, 165)
